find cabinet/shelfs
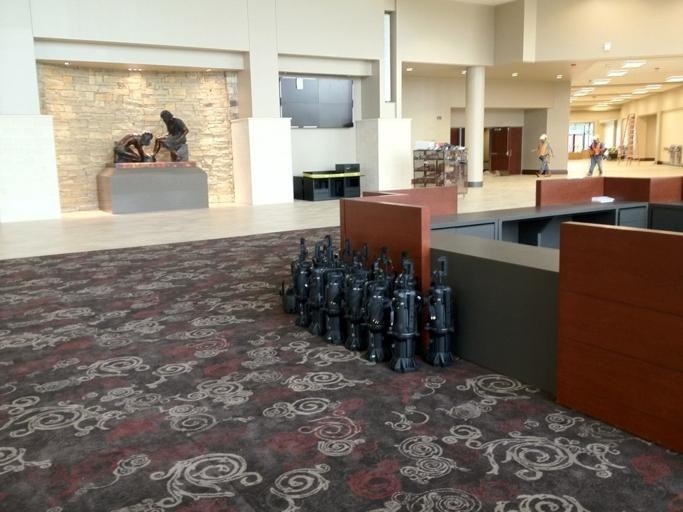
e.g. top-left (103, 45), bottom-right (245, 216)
top-left (412, 148), bottom-right (468, 198)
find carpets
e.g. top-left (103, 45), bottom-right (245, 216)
top-left (0, 225), bottom-right (683, 512)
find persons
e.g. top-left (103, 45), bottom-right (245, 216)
top-left (586, 135), bottom-right (606, 177)
top-left (113, 132), bottom-right (153, 162)
top-left (531, 134), bottom-right (552, 178)
top-left (151, 110), bottom-right (189, 163)
